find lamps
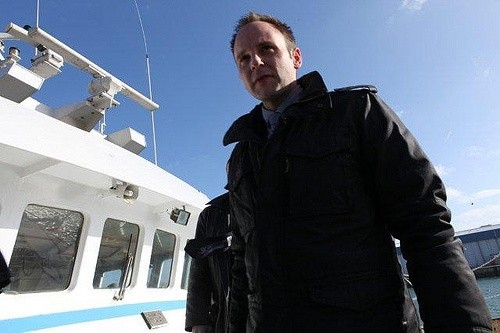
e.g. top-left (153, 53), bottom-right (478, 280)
top-left (163, 205), bottom-right (191, 226)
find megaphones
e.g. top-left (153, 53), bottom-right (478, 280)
top-left (109, 183), bottom-right (139, 204)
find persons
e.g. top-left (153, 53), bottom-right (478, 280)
top-left (223, 12), bottom-right (494, 333)
top-left (183, 183), bottom-right (248, 333)
